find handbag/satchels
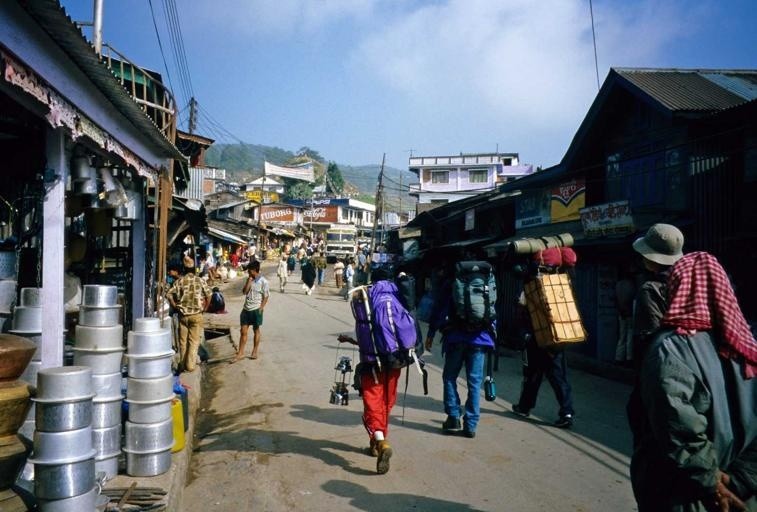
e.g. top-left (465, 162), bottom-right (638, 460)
top-left (532, 246), bottom-right (577, 267)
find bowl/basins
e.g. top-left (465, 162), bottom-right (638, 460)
top-left (9, 285), bottom-right (43, 497)
top-left (122, 316), bottom-right (176, 478)
top-left (70, 283), bottom-right (126, 483)
top-left (25, 365), bottom-right (101, 512)
top-left (0, 251), bottom-right (21, 333)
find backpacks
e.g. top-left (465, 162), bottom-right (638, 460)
top-left (352, 281), bottom-right (424, 375)
top-left (445, 260), bottom-right (498, 334)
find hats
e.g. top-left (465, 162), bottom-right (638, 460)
top-left (632, 224), bottom-right (685, 265)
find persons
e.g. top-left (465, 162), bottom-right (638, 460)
top-left (350, 267), bottom-right (403, 475)
top-left (614, 277), bottom-right (638, 365)
top-left (165, 267), bottom-right (212, 374)
top-left (167, 238), bottom-right (366, 314)
top-left (424, 259), bottom-right (498, 437)
top-left (625, 221), bottom-right (687, 454)
top-left (630, 249), bottom-right (757, 512)
top-left (511, 304), bottom-right (575, 428)
top-left (229, 261), bottom-right (269, 363)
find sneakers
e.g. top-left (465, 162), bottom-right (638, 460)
top-left (553, 417), bottom-right (574, 428)
top-left (459, 430), bottom-right (476, 438)
top-left (369, 439), bottom-right (377, 455)
top-left (376, 440), bottom-right (392, 475)
top-left (512, 404), bottom-right (529, 417)
top-left (443, 416), bottom-right (461, 430)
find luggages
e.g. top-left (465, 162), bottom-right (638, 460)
top-left (524, 274), bottom-right (587, 350)
